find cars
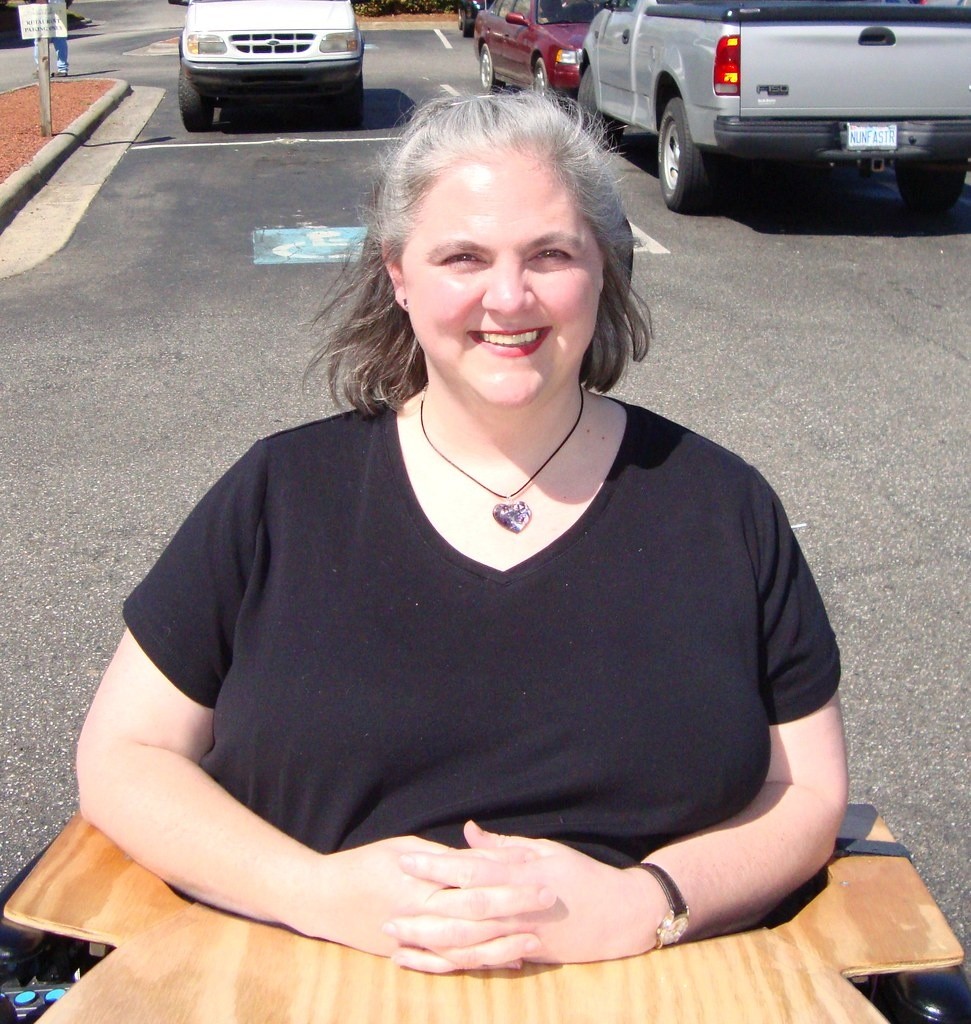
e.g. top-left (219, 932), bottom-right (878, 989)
top-left (457, 0), bottom-right (495, 38)
top-left (473, 0), bottom-right (604, 104)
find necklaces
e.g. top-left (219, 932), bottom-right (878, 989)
top-left (421, 380), bottom-right (585, 533)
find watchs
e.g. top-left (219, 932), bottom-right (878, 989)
top-left (632, 861), bottom-right (691, 949)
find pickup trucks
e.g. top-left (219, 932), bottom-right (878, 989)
top-left (578, 0), bottom-right (971, 217)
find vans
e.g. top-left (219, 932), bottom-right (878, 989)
top-left (169, 0), bottom-right (366, 134)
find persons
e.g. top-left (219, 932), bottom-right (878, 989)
top-left (76, 92), bottom-right (850, 974)
top-left (32, 0), bottom-right (69, 77)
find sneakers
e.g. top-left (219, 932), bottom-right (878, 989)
top-left (56, 73), bottom-right (67, 77)
top-left (32, 70), bottom-right (39, 79)
